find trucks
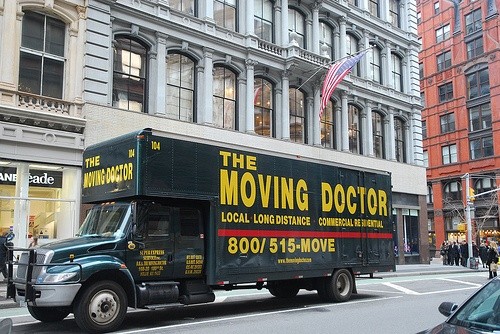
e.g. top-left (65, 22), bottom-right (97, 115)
top-left (3, 127), bottom-right (396, 334)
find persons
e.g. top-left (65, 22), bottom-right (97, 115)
top-left (0, 233), bottom-right (39, 282)
top-left (440, 240), bottom-right (500, 279)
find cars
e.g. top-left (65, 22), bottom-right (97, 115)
top-left (412, 274), bottom-right (500, 334)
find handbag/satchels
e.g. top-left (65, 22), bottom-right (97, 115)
top-left (490, 262), bottom-right (497, 272)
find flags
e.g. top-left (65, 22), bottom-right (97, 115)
top-left (318, 50), bottom-right (369, 118)
